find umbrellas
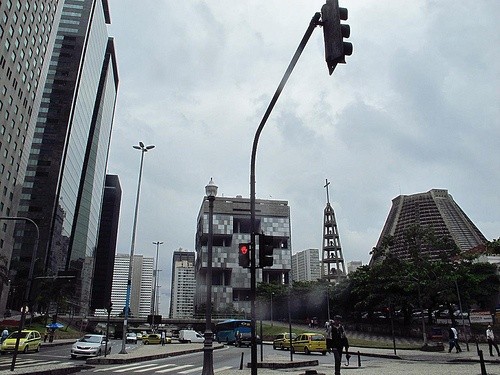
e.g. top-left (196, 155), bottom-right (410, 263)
top-left (46, 322), bottom-right (64, 329)
top-left (329, 320), bottom-right (335, 323)
top-left (312, 317), bottom-right (317, 319)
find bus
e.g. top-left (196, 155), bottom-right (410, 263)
top-left (213, 318), bottom-right (257, 345)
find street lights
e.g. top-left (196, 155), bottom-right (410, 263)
top-left (152, 241), bottom-right (164, 334)
top-left (200, 177), bottom-right (220, 375)
top-left (119, 141), bottom-right (156, 354)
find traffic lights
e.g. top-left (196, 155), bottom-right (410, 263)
top-left (259, 233), bottom-right (274, 267)
top-left (320, 0), bottom-right (353, 76)
top-left (239, 243), bottom-right (249, 266)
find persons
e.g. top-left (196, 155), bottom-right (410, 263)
top-left (486, 325), bottom-right (500, 357)
top-left (324, 315), bottom-right (350, 375)
top-left (1, 326), bottom-right (9, 342)
top-left (448, 324), bottom-right (462, 353)
top-left (43, 328), bottom-right (55, 343)
top-left (309, 320), bottom-right (319, 329)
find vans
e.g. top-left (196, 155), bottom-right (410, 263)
top-left (178, 329), bottom-right (205, 343)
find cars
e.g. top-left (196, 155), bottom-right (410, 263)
top-left (69, 333), bottom-right (112, 361)
top-left (358, 302), bottom-right (477, 321)
top-left (292, 332), bottom-right (328, 356)
top-left (142, 333), bottom-right (171, 346)
top-left (112, 323), bottom-right (180, 340)
top-left (0, 328), bottom-right (42, 355)
top-left (125, 332), bottom-right (138, 344)
top-left (273, 331), bottom-right (298, 351)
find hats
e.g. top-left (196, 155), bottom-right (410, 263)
top-left (334, 315), bottom-right (342, 321)
top-left (488, 325), bottom-right (491, 328)
